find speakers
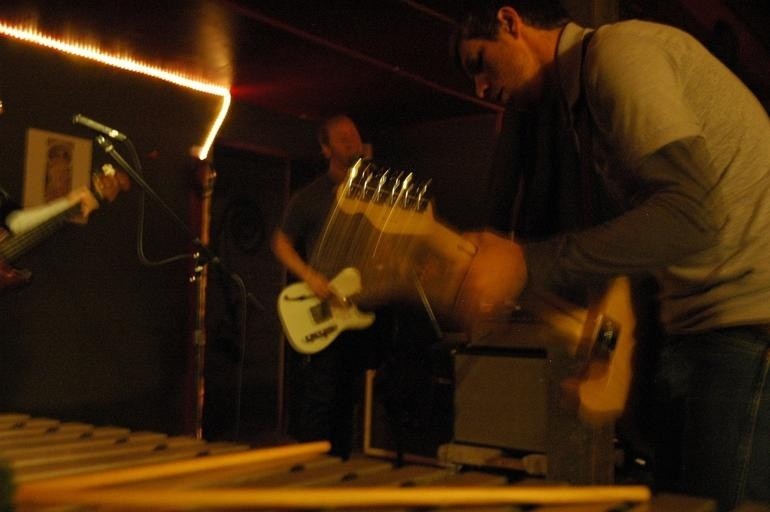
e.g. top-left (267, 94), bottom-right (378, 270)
top-left (451, 349), bottom-right (548, 454)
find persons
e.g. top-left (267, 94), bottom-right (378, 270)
top-left (451, 0), bottom-right (770, 511)
top-left (264, 111), bottom-right (433, 463)
top-left (0, 165), bottom-right (131, 253)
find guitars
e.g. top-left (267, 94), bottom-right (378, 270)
top-left (1, 165), bottom-right (131, 282)
top-left (278, 265), bottom-right (404, 352)
top-left (335, 159), bottom-right (634, 412)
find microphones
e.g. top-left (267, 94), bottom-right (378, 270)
top-left (62, 110), bottom-right (127, 142)
top-left (351, 153), bottom-right (380, 174)
